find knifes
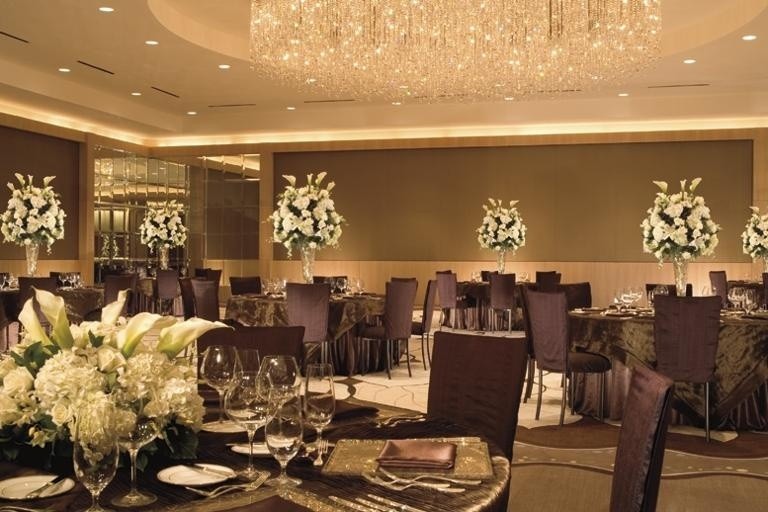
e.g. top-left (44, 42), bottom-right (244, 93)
top-left (173, 458), bottom-right (234, 477)
top-left (327, 486), bottom-right (434, 512)
top-left (27, 471), bottom-right (70, 498)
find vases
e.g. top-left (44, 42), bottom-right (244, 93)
top-left (295, 240), bottom-right (316, 285)
top-left (498, 248), bottom-right (506, 274)
top-left (22, 237), bottom-right (41, 277)
top-left (157, 244), bottom-right (172, 271)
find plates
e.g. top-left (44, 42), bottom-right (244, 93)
top-left (161, 461), bottom-right (234, 490)
top-left (3, 472), bottom-right (81, 500)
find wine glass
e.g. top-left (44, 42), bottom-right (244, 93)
top-left (128, 265), bottom-right (189, 278)
top-left (266, 355), bottom-right (298, 428)
top-left (266, 395), bottom-right (303, 487)
top-left (613, 284), bottom-right (766, 322)
top-left (260, 274), bottom-right (369, 298)
top-left (304, 365), bottom-right (332, 460)
top-left (205, 343), bottom-right (262, 400)
top-left (231, 376), bottom-right (273, 481)
top-left (2, 271), bottom-right (84, 292)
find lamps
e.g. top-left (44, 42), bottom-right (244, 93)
top-left (247, 1), bottom-right (662, 106)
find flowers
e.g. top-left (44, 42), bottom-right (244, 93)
top-left (0, 173), bottom-right (66, 257)
top-left (743, 206), bottom-right (768, 264)
top-left (640, 179), bottom-right (721, 263)
top-left (262, 172), bottom-right (348, 260)
top-left (472, 198), bottom-right (529, 257)
top-left (139, 200), bottom-right (190, 251)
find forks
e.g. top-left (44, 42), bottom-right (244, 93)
top-left (369, 466), bottom-right (490, 495)
